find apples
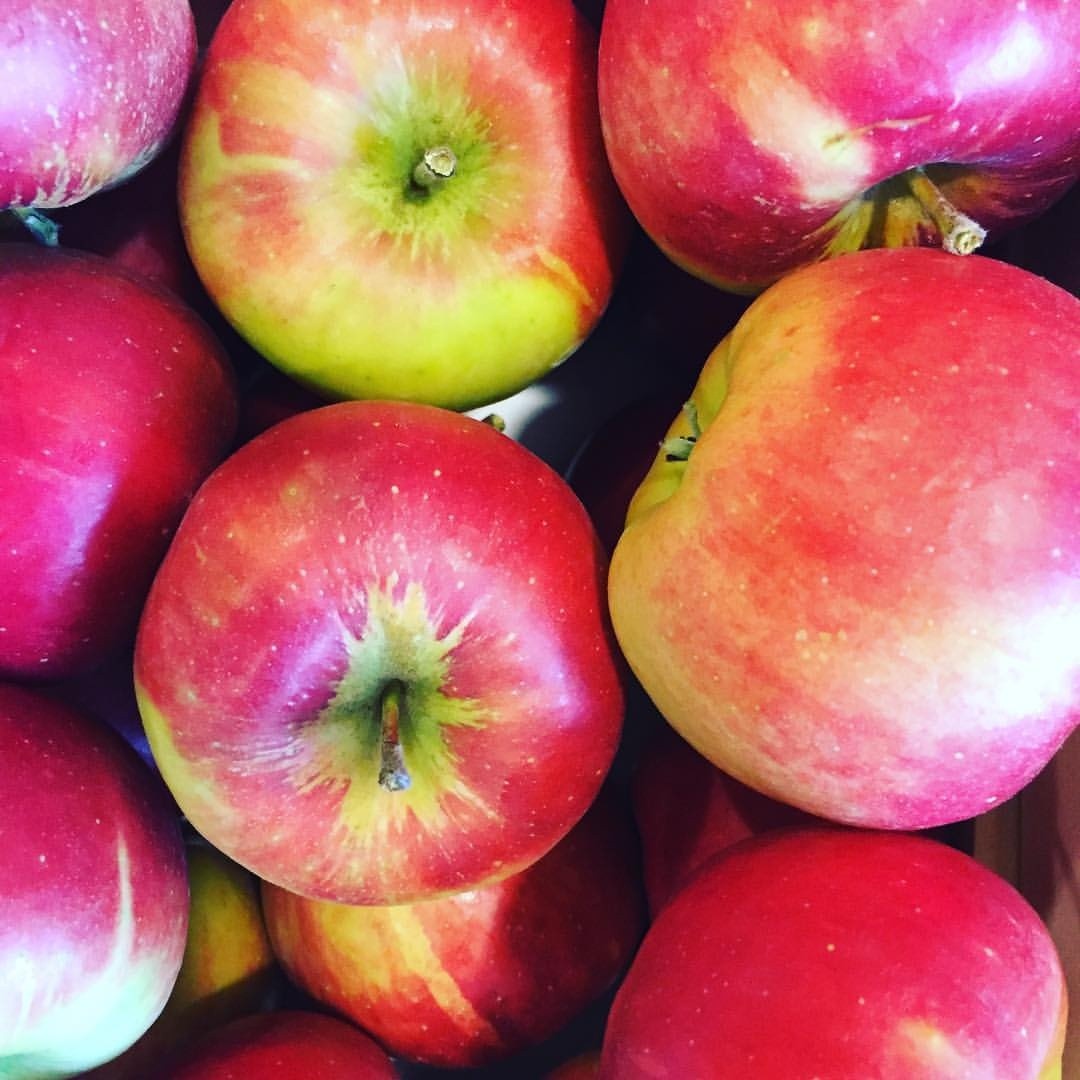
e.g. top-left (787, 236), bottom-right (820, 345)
top-left (0, 0), bottom-right (1080, 1079)
